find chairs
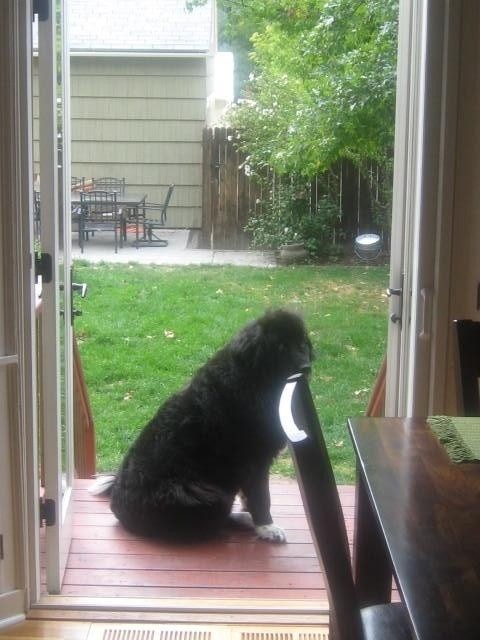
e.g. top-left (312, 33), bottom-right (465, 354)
top-left (275, 363), bottom-right (415, 640)
top-left (453, 318), bottom-right (480, 417)
top-left (33, 174), bottom-right (177, 254)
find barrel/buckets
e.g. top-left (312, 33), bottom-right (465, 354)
top-left (354, 232), bottom-right (382, 267)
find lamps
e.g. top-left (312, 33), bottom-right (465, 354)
top-left (351, 233), bottom-right (387, 267)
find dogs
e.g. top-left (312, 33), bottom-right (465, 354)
top-left (88, 309), bottom-right (315, 547)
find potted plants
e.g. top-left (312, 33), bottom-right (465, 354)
top-left (239, 190), bottom-right (345, 267)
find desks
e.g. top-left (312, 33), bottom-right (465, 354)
top-left (344, 412), bottom-right (480, 640)
